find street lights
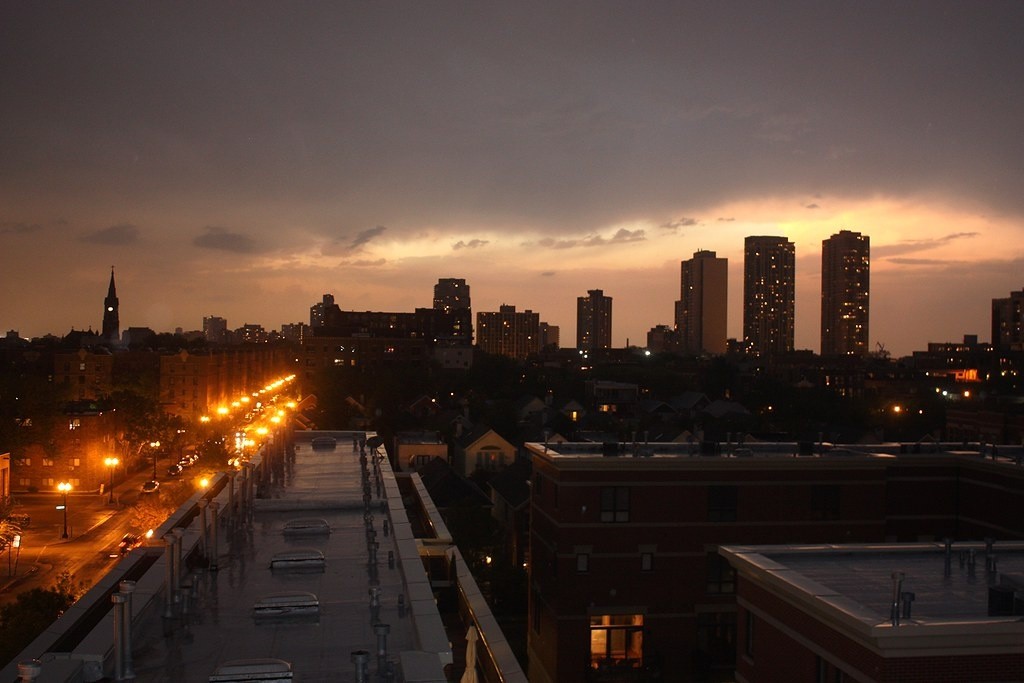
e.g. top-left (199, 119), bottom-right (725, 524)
top-left (104, 458), bottom-right (118, 504)
top-left (150, 440), bottom-right (160, 479)
top-left (58, 482), bottom-right (70, 539)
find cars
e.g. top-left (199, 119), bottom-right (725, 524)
top-left (139, 453), bottom-right (202, 495)
